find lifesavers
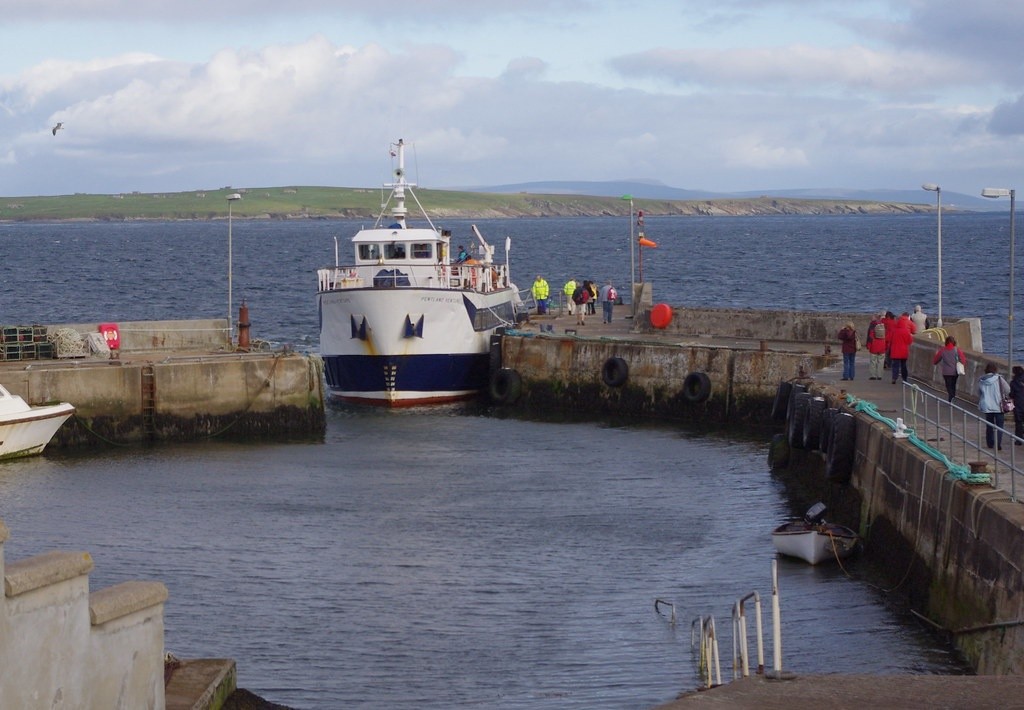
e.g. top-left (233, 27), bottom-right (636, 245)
top-left (602, 356), bottom-right (628, 387)
top-left (488, 368), bottom-right (522, 403)
top-left (684, 372), bottom-right (711, 402)
top-left (770, 380), bottom-right (858, 481)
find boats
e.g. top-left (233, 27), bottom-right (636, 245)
top-left (1, 381), bottom-right (78, 463)
top-left (313, 139), bottom-right (533, 406)
top-left (770, 517), bottom-right (858, 567)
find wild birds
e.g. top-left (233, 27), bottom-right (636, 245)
top-left (52, 122), bottom-right (65, 136)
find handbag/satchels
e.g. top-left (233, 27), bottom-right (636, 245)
top-left (608, 287), bottom-right (616, 300)
top-left (1001, 396), bottom-right (1015, 413)
top-left (855, 332), bottom-right (861, 351)
top-left (956, 362), bottom-right (965, 376)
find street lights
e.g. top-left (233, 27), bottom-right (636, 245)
top-left (225, 193), bottom-right (241, 345)
top-left (981, 186), bottom-right (1017, 383)
top-left (920, 184), bottom-right (942, 329)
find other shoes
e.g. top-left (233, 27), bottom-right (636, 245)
top-left (1015, 441), bottom-right (1023, 445)
top-left (869, 377), bottom-right (875, 380)
top-left (878, 378), bottom-right (881, 380)
top-left (892, 379), bottom-right (896, 384)
top-left (998, 445), bottom-right (1001, 450)
top-left (850, 378), bottom-right (853, 380)
top-left (577, 322), bottom-right (579, 325)
top-left (842, 378), bottom-right (848, 380)
top-left (581, 321), bottom-right (584, 325)
top-left (989, 445), bottom-right (993, 448)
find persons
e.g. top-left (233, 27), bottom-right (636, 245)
top-left (572, 282), bottom-right (587, 325)
top-left (532, 275), bottom-right (549, 313)
top-left (456, 246), bottom-right (465, 261)
top-left (866, 314), bottom-right (887, 381)
top-left (563, 279), bottom-right (599, 315)
top-left (599, 280), bottom-right (616, 324)
top-left (932, 336), bottom-right (966, 407)
top-left (391, 248), bottom-right (405, 259)
top-left (875, 306), bottom-right (929, 384)
top-left (1009, 365), bottom-right (1024, 445)
top-left (838, 322), bottom-right (857, 381)
top-left (977, 363), bottom-right (1010, 450)
top-left (463, 255), bottom-right (472, 261)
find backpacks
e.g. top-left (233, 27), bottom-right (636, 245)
top-left (874, 322), bottom-right (886, 338)
